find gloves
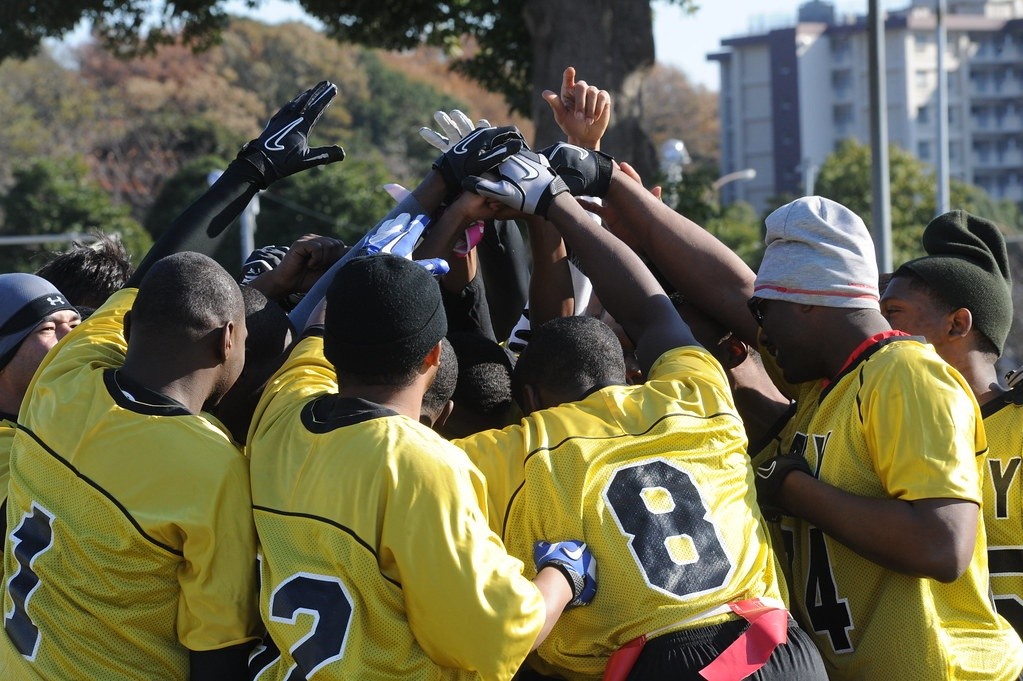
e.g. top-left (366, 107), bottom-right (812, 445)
top-left (989, 366), bottom-right (1023, 407)
top-left (420, 109), bottom-right (493, 154)
top-left (534, 538), bottom-right (598, 610)
top-left (236, 80), bottom-right (345, 190)
top-left (361, 212), bottom-right (450, 276)
top-left (461, 146), bottom-right (570, 221)
top-left (756, 454), bottom-right (813, 508)
top-left (433, 125), bottom-right (531, 194)
top-left (536, 142), bottom-right (614, 199)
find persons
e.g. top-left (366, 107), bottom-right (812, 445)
top-left (0, 67), bottom-right (1023, 681)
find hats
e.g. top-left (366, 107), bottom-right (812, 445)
top-left (236, 245), bottom-right (307, 305)
top-left (0, 272), bottom-right (82, 366)
top-left (753, 195), bottom-right (881, 310)
top-left (322, 253), bottom-right (449, 371)
top-left (902, 210), bottom-right (1014, 359)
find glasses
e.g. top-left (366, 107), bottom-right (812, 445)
top-left (747, 293), bottom-right (767, 329)
